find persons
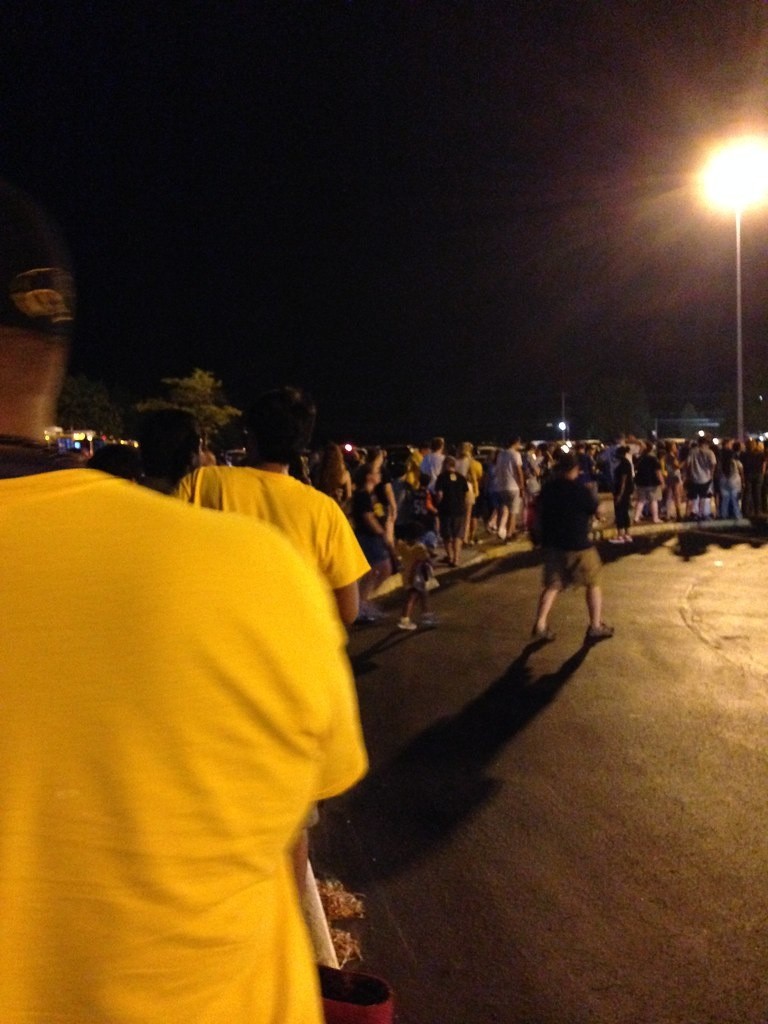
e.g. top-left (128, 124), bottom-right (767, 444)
top-left (0, 182), bottom-right (368, 1024)
top-left (529, 455), bottom-right (616, 640)
top-left (170, 383), bottom-right (373, 626)
top-left (434, 456), bottom-right (470, 566)
top-left (350, 465), bottom-right (392, 621)
top-left (394, 474), bottom-right (438, 630)
top-left (608, 446), bottom-right (635, 544)
top-left (291, 436), bottom-right (768, 573)
top-left (86, 408), bottom-right (203, 495)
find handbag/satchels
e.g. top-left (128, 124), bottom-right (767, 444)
top-left (526, 476), bottom-right (540, 494)
top-left (413, 562), bottom-right (439, 591)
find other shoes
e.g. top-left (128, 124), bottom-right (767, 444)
top-left (462, 520), bottom-right (528, 549)
top-left (397, 616), bottom-right (417, 631)
top-left (683, 512), bottom-right (696, 522)
top-left (608, 536), bottom-right (625, 544)
top-left (355, 611), bottom-right (375, 623)
top-left (437, 556), bottom-right (450, 563)
top-left (360, 604), bottom-right (390, 618)
top-left (419, 615), bottom-right (437, 632)
top-left (448, 563), bottom-right (457, 568)
top-left (693, 513), bottom-right (709, 521)
top-left (623, 535), bottom-right (633, 542)
top-left (587, 623), bottom-right (613, 639)
top-left (533, 623), bottom-right (557, 641)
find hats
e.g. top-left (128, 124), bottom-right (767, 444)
top-left (412, 542), bottom-right (426, 554)
top-left (0, 182), bottom-right (77, 335)
